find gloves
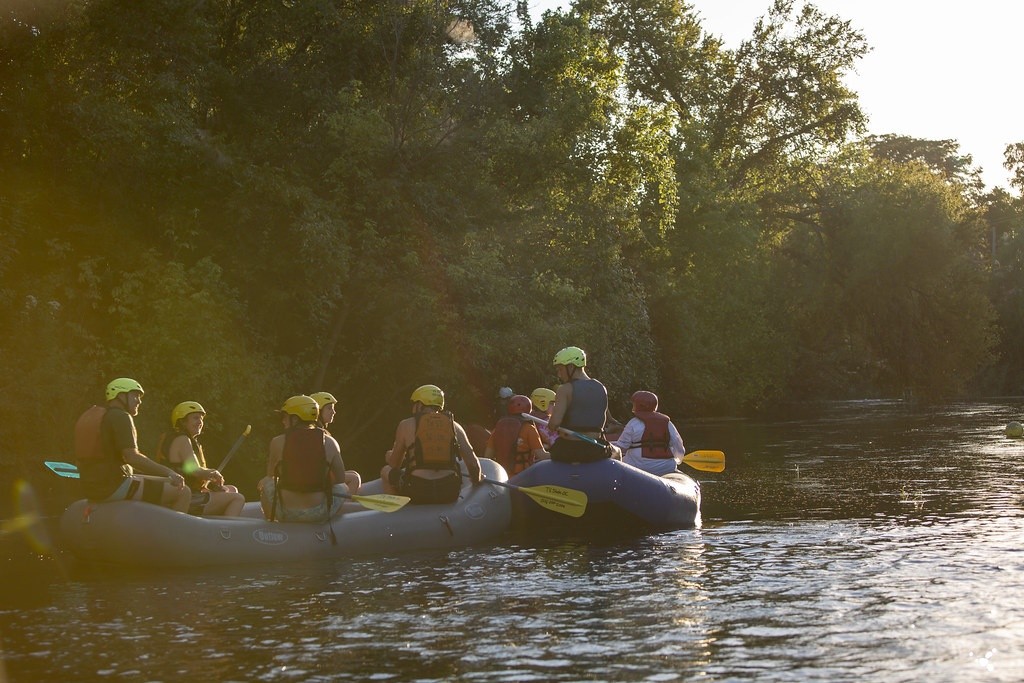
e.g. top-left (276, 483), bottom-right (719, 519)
top-left (499, 387), bottom-right (513, 398)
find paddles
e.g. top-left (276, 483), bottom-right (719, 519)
top-left (521, 412), bottom-right (608, 449)
top-left (459, 471), bottom-right (588, 518)
top-left (218, 424), bottom-right (252, 472)
top-left (681, 449), bottom-right (726, 472)
top-left (43, 461), bottom-right (185, 489)
top-left (334, 492), bottom-right (411, 512)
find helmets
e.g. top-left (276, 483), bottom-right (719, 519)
top-left (411, 384), bottom-right (445, 411)
top-left (282, 395), bottom-right (319, 422)
top-left (508, 395), bottom-right (531, 415)
top-left (172, 401), bottom-right (206, 428)
top-left (531, 387), bottom-right (556, 411)
top-left (631, 390), bottom-right (658, 411)
top-left (310, 391), bottom-right (338, 410)
top-left (553, 346), bottom-right (587, 367)
top-left (105, 378), bottom-right (144, 402)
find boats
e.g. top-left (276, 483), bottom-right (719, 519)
top-left (512, 453), bottom-right (702, 532)
top-left (59, 456), bottom-right (512, 568)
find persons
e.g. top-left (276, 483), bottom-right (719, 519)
top-left (256, 395), bottom-right (349, 524)
top-left (75, 377), bottom-right (191, 514)
top-left (615, 391), bottom-right (686, 476)
top-left (486, 395), bottom-right (550, 479)
top-left (309, 392), bottom-right (362, 497)
top-left (155, 402), bottom-right (245, 518)
top-left (530, 388), bottom-right (558, 451)
top-left (548, 346), bottom-right (623, 463)
top-left (382, 384), bottom-right (485, 505)
